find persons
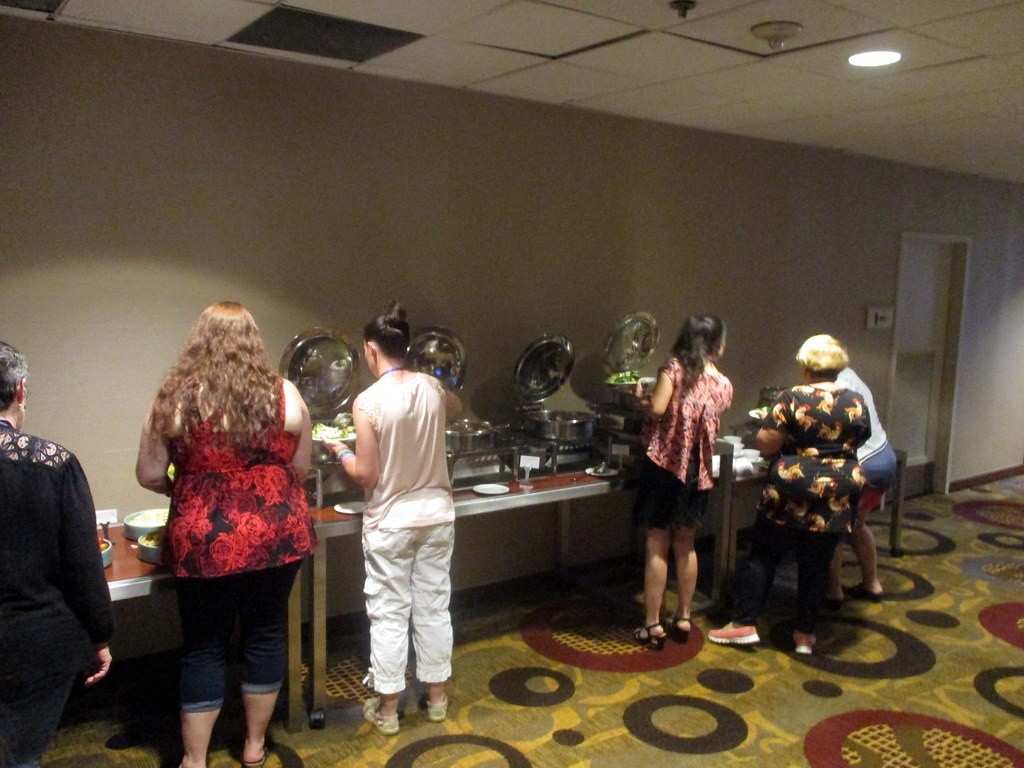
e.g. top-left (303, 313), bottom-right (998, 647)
top-left (821, 366), bottom-right (897, 612)
top-left (707, 333), bottom-right (871, 654)
top-left (629, 314), bottom-right (733, 651)
top-left (320, 299), bottom-right (463, 736)
top-left (0, 341), bottom-right (116, 768)
top-left (135, 301), bottom-right (312, 768)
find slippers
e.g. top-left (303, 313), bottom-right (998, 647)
top-left (239, 740), bottom-right (265, 768)
top-left (846, 583), bottom-right (881, 603)
top-left (823, 595), bottom-right (846, 610)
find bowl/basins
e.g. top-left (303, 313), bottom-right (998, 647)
top-left (123, 508), bottom-right (169, 541)
top-left (723, 436), bottom-right (763, 465)
top-left (97, 536), bottom-right (113, 568)
top-left (136, 532), bottom-right (166, 564)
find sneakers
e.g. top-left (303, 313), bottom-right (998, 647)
top-left (363, 697), bottom-right (399, 735)
top-left (426, 692), bottom-right (448, 722)
top-left (793, 629), bottom-right (816, 654)
top-left (708, 621), bottom-right (760, 644)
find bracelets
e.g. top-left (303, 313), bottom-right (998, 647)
top-left (334, 445), bottom-right (357, 466)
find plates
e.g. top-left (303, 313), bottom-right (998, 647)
top-left (311, 427), bottom-right (357, 443)
top-left (584, 467), bottom-right (618, 476)
top-left (473, 483), bottom-right (509, 494)
top-left (605, 379), bottom-right (637, 385)
top-left (334, 501), bottom-right (364, 514)
top-left (748, 408), bottom-right (770, 419)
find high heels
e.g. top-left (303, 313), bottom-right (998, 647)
top-left (633, 624), bottom-right (666, 651)
top-left (664, 616), bottom-right (691, 643)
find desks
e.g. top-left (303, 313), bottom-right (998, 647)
top-left (98, 438), bottom-right (908, 735)
